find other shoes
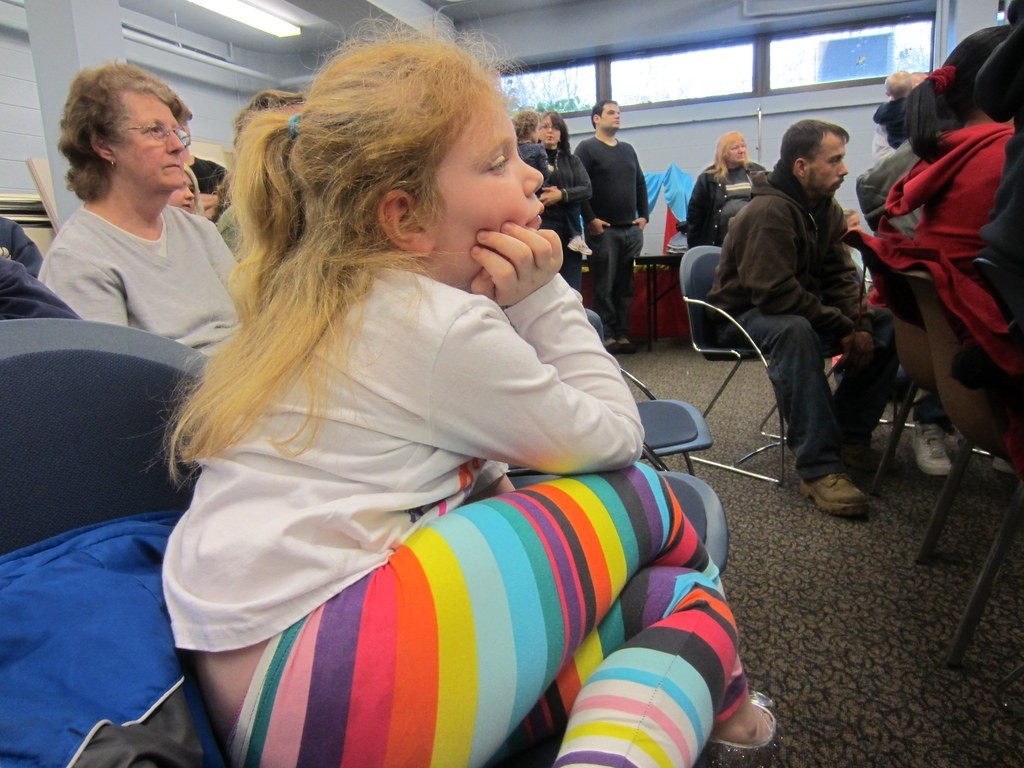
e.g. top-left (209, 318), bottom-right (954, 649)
top-left (703, 691), bottom-right (781, 768)
top-left (604, 335), bottom-right (636, 355)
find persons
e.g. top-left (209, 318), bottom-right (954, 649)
top-left (685, 0), bottom-right (1024, 518)
top-left (0, 63), bottom-right (650, 359)
top-left (161, 30), bottom-right (776, 768)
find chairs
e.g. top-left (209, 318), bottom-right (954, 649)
top-left (680, 245), bottom-right (795, 486)
top-left (581, 307), bottom-right (713, 477)
top-left (854, 249), bottom-right (1024, 670)
top-left (0, 317), bottom-right (211, 562)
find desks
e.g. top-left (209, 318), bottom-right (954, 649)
top-left (635, 253), bottom-right (684, 352)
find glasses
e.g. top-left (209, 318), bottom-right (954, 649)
top-left (127, 122), bottom-right (191, 146)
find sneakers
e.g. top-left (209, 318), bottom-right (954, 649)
top-left (568, 236), bottom-right (593, 255)
top-left (991, 456), bottom-right (1017, 476)
top-left (911, 423), bottom-right (953, 476)
top-left (799, 473), bottom-right (871, 517)
top-left (841, 442), bottom-right (874, 472)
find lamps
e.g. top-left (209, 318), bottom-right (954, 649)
top-left (187, 0), bottom-right (302, 38)
